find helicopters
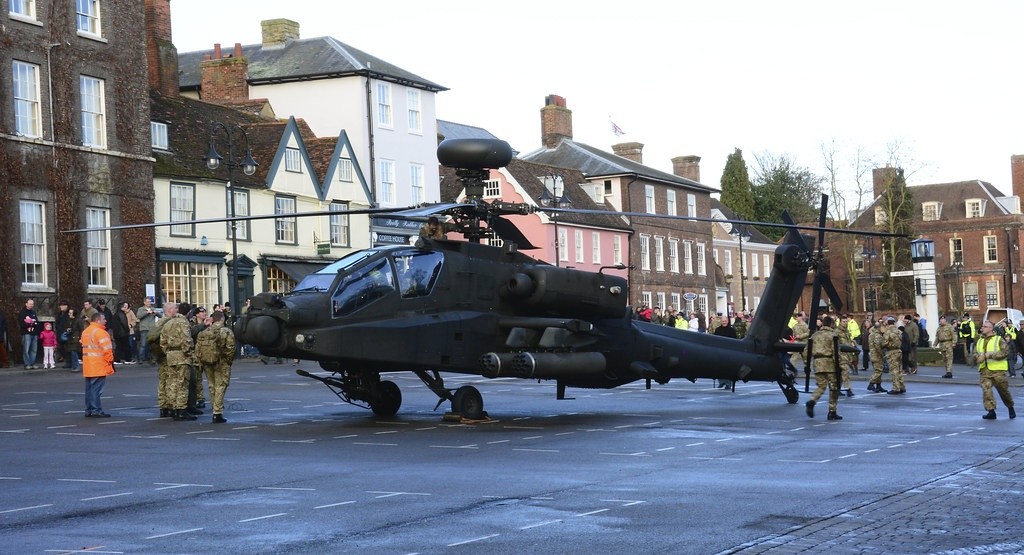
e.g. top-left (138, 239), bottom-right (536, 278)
top-left (60, 138), bottom-right (911, 421)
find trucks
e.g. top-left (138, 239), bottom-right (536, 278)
top-left (982, 305), bottom-right (1024, 331)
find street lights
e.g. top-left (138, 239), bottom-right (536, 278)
top-left (538, 171), bottom-right (572, 267)
top-left (201, 121), bottom-right (260, 359)
top-left (728, 214), bottom-right (753, 310)
top-left (950, 259), bottom-right (966, 316)
top-left (860, 235), bottom-right (877, 313)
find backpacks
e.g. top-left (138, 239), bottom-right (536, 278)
top-left (197, 326), bottom-right (224, 365)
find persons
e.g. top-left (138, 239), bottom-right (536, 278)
top-left (834, 315), bottom-right (861, 398)
top-left (240, 298), bottom-right (301, 366)
top-left (146, 302), bottom-right (214, 421)
top-left (802, 316), bottom-right (842, 421)
top-left (788, 313), bottom-right (929, 374)
top-left (932, 315), bottom-right (958, 380)
top-left (714, 317), bottom-right (738, 389)
top-left (194, 311), bottom-right (236, 424)
top-left (631, 305), bottom-right (755, 339)
top-left (415, 216), bottom-right (443, 249)
top-left (950, 313), bottom-right (1024, 378)
top-left (18, 296), bottom-right (236, 369)
top-left (972, 320), bottom-right (1016, 420)
top-left (866, 317), bottom-right (906, 395)
top-left (79, 313), bottom-right (116, 418)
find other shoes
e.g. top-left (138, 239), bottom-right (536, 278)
top-left (160, 401), bottom-right (205, 421)
top-left (806, 401), bottom-right (814, 417)
top-left (887, 390), bottom-right (906, 394)
top-left (85, 412), bottom-right (111, 417)
top-left (983, 411), bottom-right (996, 419)
top-left (828, 412), bottom-right (842, 420)
top-left (942, 372), bottom-right (952, 378)
top-left (212, 414), bottom-right (227, 423)
top-left (868, 383), bottom-right (887, 392)
top-left (1009, 407), bottom-right (1016, 418)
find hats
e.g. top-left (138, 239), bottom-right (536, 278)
top-left (888, 317), bottom-right (894, 320)
top-left (225, 302), bottom-right (230, 307)
top-left (867, 312), bottom-right (872, 316)
top-left (939, 315), bottom-right (945, 322)
top-left (905, 315), bottom-right (911, 320)
